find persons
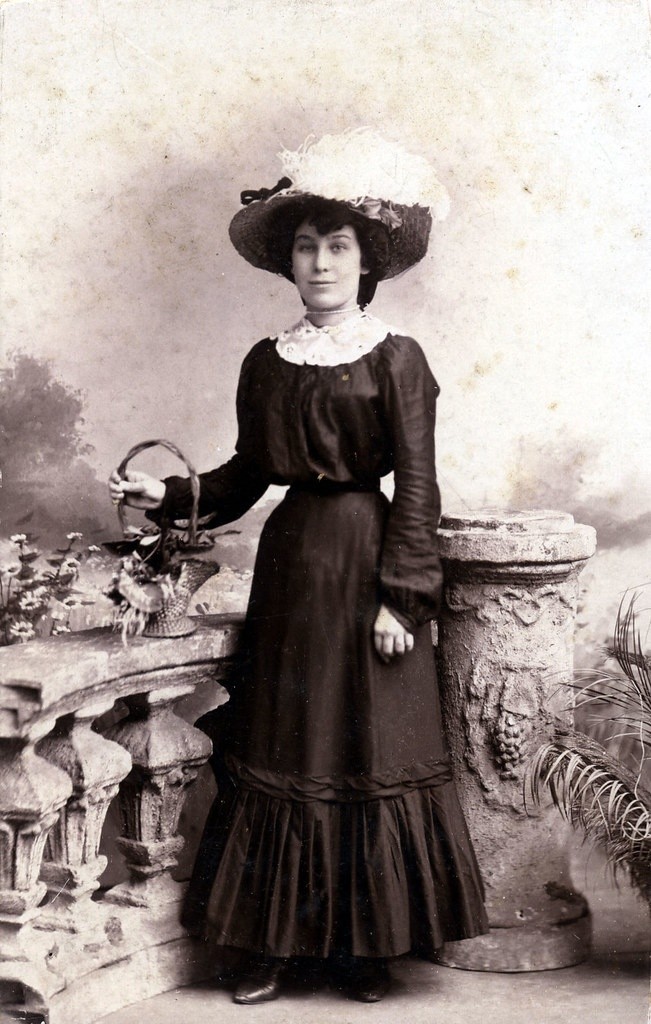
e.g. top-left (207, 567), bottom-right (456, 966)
top-left (107, 126), bottom-right (495, 1005)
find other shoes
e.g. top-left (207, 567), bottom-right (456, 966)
top-left (233, 959), bottom-right (291, 1004)
top-left (358, 966), bottom-right (389, 1001)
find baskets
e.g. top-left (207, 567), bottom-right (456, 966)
top-left (103, 437), bottom-right (220, 637)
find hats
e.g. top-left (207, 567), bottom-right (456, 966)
top-left (229, 127), bottom-right (450, 281)
